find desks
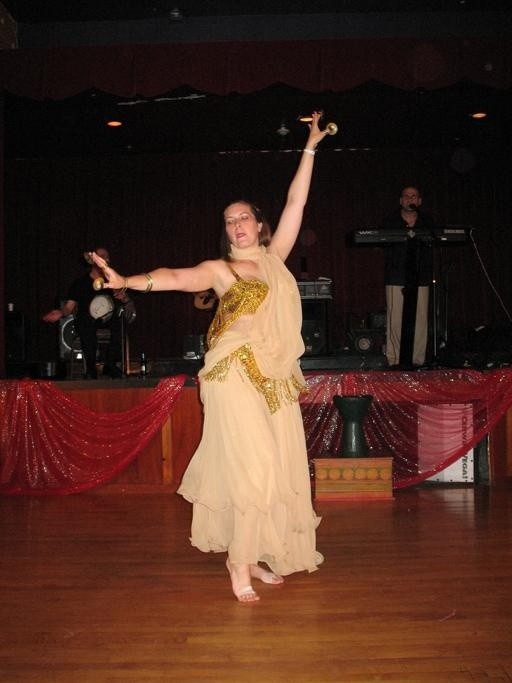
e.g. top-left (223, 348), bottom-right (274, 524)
top-left (6, 368), bottom-right (512, 488)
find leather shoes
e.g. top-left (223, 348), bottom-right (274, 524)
top-left (86, 366), bottom-right (98, 379)
top-left (102, 366), bottom-right (123, 378)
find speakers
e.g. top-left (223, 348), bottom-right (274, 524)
top-left (302, 300), bottom-right (328, 356)
top-left (60, 314), bottom-right (100, 359)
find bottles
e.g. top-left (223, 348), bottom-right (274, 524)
top-left (140, 353), bottom-right (147, 375)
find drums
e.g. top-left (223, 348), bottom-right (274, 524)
top-left (89, 296), bottom-right (136, 324)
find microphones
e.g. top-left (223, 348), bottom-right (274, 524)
top-left (409, 203), bottom-right (417, 210)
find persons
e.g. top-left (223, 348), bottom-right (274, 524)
top-left (41, 247), bottom-right (129, 381)
top-left (376, 187), bottom-right (443, 370)
top-left (88, 111), bottom-right (326, 602)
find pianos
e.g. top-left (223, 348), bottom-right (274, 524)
top-left (355, 229), bottom-right (469, 245)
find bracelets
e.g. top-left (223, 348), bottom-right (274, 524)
top-left (305, 148), bottom-right (317, 158)
top-left (118, 274), bottom-right (129, 294)
top-left (140, 269), bottom-right (154, 294)
top-left (60, 308), bottom-right (66, 319)
